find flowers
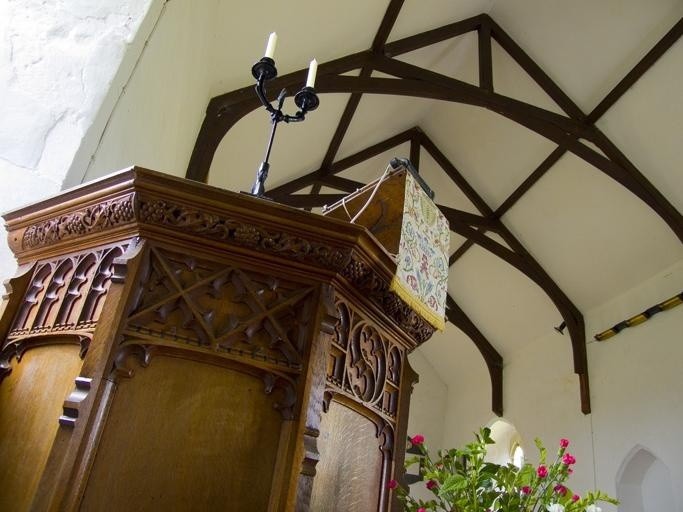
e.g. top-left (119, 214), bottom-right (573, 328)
top-left (380, 425), bottom-right (584, 510)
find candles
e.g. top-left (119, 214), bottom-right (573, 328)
top-left (264, 30), bottom-right (278, 57)
top-left (306, 56), bottom-right (319, 90)
